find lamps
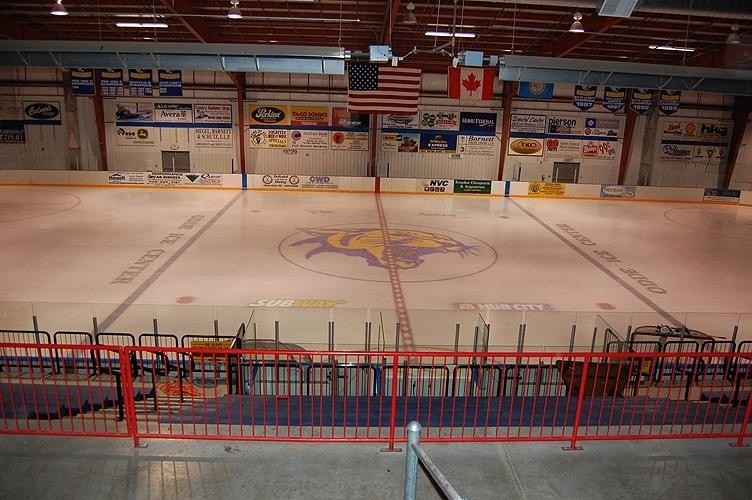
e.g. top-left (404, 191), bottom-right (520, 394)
top-left (726, 25), bottom-right (741, 44)
top-left (402, 1), bottom-right (417, 24)
top-left (424, 31), bottom-right (476, 38)
top-left (50, 0), bottom-right (69, 16)
top-left (227, 0), bottom-right (243, 18)
top-left (648, 43), bottom-right (695, 52)
top-left (115, 22), bottom-right (168, 28)
top-left (568, 12), bottom-right (585, 32)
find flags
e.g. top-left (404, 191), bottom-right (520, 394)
top-left (347, 63), bottom-right (422, 115)
top-left (448, 66), bottom-right (496, 100)
